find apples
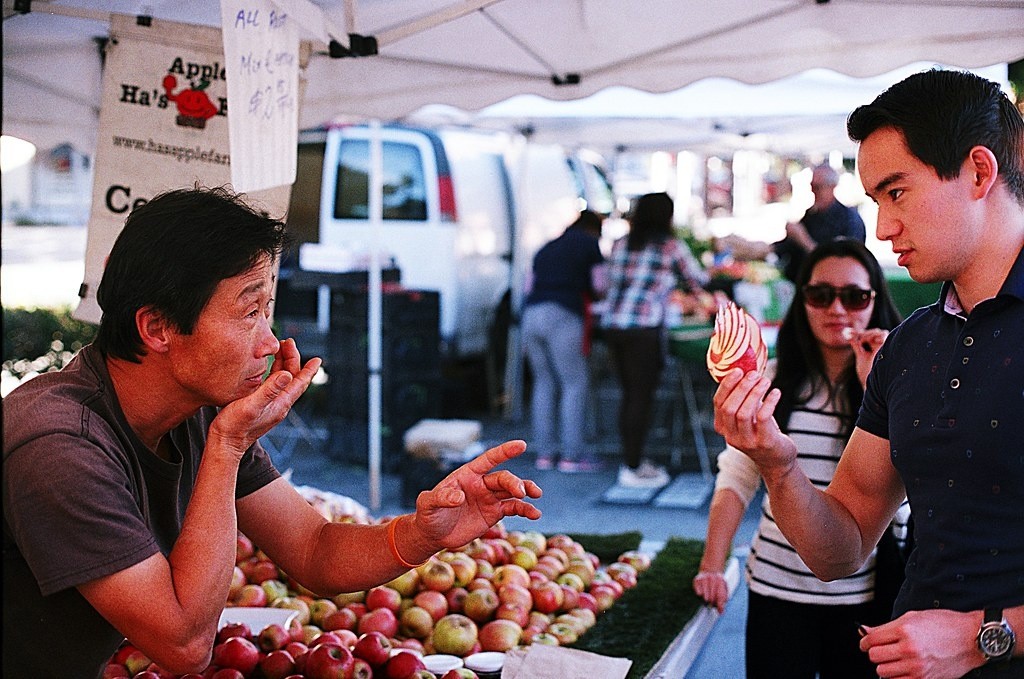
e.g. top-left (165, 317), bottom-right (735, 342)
top-left (706, 301), bottom-right (768, 387)
top-left (96, 513), bottom-right (651, 679)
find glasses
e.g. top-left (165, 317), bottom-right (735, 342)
top-left (801, 283), bottom-right (878, 310)
top-left (811, 181), bottom-right (826, 190)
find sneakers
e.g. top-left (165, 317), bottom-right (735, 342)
top-left (618, 458), bottom-right (670, 488)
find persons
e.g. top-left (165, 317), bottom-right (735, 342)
top-left (518, 209), bottom-right (607, 475)
top-left (600, 193), bottom-right (711, 488)
top-left (712, 69), bottom-right (1024, 679)
top-left (0, 188), bottom-right (542, 679)
top-left (776, 164), bottom-right (867, 284)
top-left (694, 237), bottom-right (904, 679)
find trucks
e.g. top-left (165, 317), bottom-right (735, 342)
top-left (266, 122), bottom-right (617, 464)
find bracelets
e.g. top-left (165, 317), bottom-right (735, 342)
top-left (389, 514), bottom-right (429, 568)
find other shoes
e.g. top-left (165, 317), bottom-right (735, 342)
top-left (533, 454), bottom-right (557, 470)
top-left (557, 455), bottom-right (609, 472)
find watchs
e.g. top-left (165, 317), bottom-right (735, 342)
top-left (974, 603), bottom-right (1015, 663)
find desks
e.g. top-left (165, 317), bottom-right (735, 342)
top-left (653, 320), bottom-right (783, 483)
top-left (546, 529), bottom-right (741, 679)
